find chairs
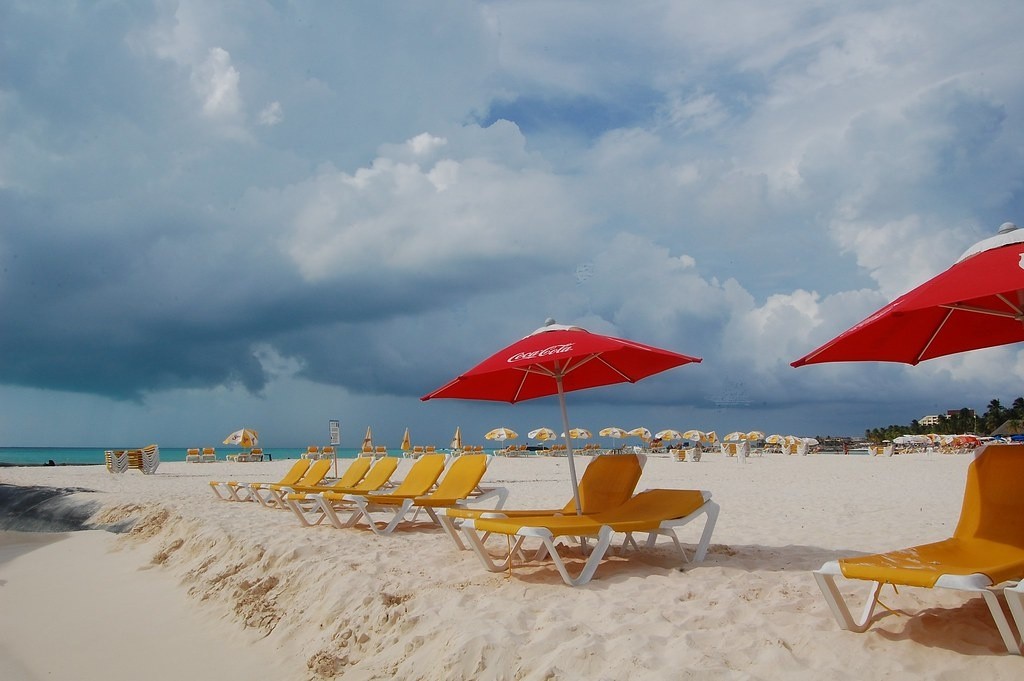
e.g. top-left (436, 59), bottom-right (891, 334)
top-left (186, 441), bottom-right (809, 585)
top-left (812, 445), bottom-right (1024, 655)
top-left (105, 445), bottom-right (164, 475)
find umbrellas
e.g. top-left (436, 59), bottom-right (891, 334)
top-left (626, 426), bottom-right (651, 448)
top-left (784, 434), bottom-right (819, 453)
top-left (561, 427), bottom-right (593, 449)
top-left (705, 431), bottom-right (720, 447)
top-left (362, 426), bottom-right (372, 449)
top-left (788, 221), bottom-right (1024, 367)
top-left (654, 429), bottom-right (682, 445)
top-left (723, 431), bottom-right (748, 443)
top-left (598, 426), bottom-right (628, 448)
top-left (401, 426), bottom-right (410, 450)
top-left (892, 433), bottom-right (1024, 448)
top-left (484, 426), bottom-right (519, 448)
top-left (223, 427), bottom-right (259, 461)
top-left (682, 429), bottom-right (707, 446)
top-left (419, 317), bottom-right (703, 555)
top-left (528, 426), bottom-right (558, 450)
top-left (747, 430), bottom-right (766, 447)
top-left (451, 425), bottom-right (462, 449)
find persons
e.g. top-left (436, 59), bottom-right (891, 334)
top-left (772, 443), bottom-right (783, 454)
top-left (651, 438), bottom-right (718, 452)
